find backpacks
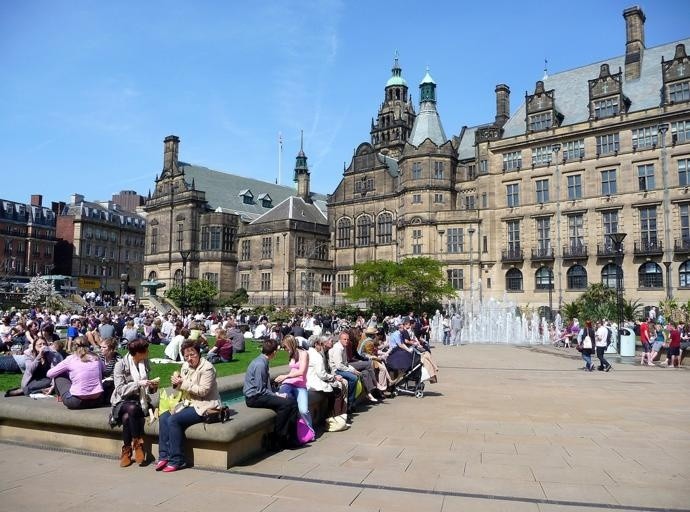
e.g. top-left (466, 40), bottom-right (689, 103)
top-left (606, 328), bottom-right (612, 345)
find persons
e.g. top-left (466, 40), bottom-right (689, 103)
top-left (1, 290), bottom-right (465, 473)
top-left (551, 306), bottom-right (690, 372)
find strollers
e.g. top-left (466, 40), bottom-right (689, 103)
top-left (389, 351), bottom-right (439, 398)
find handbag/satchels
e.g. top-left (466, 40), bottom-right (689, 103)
top-left (296, 418), bottom-right (314, 443)
top-left (202, 404), bottom-right (232, 425)
top-left (323, 413), bottom-right (348, 432)
top-left (582, 334), bottom-right (592, 350)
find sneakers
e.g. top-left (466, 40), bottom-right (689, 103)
top-left (92, 346), bottom-right (101, 353)
top-left (597, 366), bottom-right (604, 371)
top-left (648, 362), bottom-right (655, 366)
top-left (583, 367), bottom-right (589, 371)
top-left (640, 361), bottom-right (646, 365)
top-left (164, 464), bottom-right (181, 473)
top-left (590, 363), bottom-right (594, 371)
top-left (604, 364), bottom-right (611, 372)
top-left (366, 393), bottom-right (377, 403)
top-left (156, 459), bottom-right (167, 470)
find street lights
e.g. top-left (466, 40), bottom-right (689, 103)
top-left (609, 233), bottom-right (629, 331)
top-left (543, 263), bottom-right (555, 326)
top-left (661, 262), bottom-right (672, 302)
top-left (177, 249), bottom-right (193, 316)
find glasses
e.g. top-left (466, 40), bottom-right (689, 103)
top-left (182, 352), bottom-right (198, 359)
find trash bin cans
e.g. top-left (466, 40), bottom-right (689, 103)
top-left (619, 327), bottom-right (636, 357)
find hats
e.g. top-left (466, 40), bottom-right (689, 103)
top-left (365, 328), bottom-right (379, 334)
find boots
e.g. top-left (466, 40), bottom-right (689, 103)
top-left (121, 446), bottom-right (132, 467)
top-left (132, 437), bottom-right (148, 465)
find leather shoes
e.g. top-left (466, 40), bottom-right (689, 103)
top-left (5, 387), bottom-right (20, 397)
